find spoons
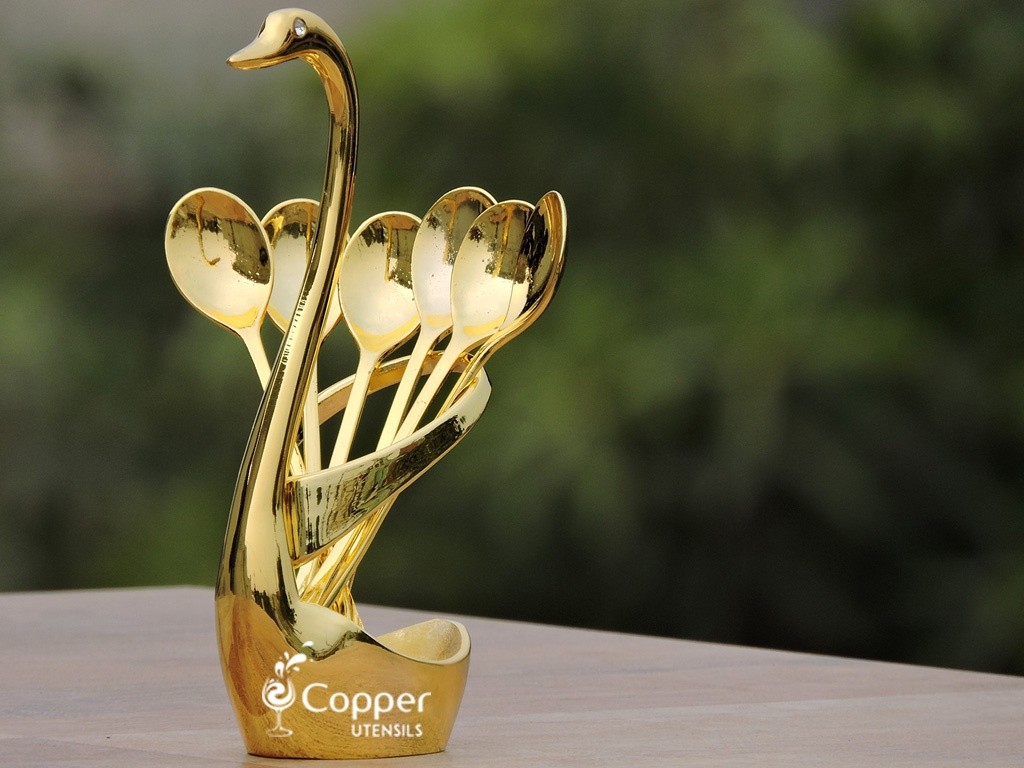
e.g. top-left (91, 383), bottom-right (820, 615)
top-left (375, 185), bottom-right (499, 452)
top-left (302, 197), bottom-right (535, 606)
top-left (296, 210), bottom-right (422, 597)
top-left (321, 189), bottom-right (568, 609)
top-left (260, 197), bottom-right (352, 473)
top-left (163, 187), bottom-right (305, 475)
